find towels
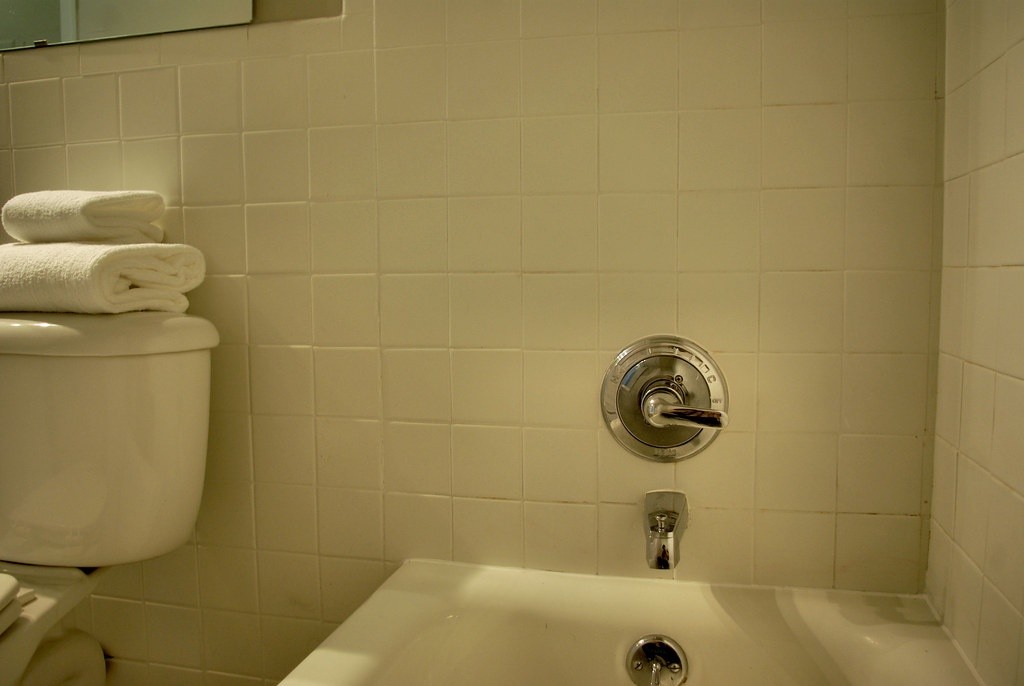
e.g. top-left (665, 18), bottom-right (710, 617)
top-left (0, 189), bottom-right (205, 313)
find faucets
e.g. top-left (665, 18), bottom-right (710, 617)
top-left (641, 489), bottom-right (691, 570)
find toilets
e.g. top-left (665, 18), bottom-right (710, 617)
top-left (0, 314), bottom-right (220, 685)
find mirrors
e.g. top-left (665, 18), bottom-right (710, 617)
top-left (0, 0), bottom-right (254, 54)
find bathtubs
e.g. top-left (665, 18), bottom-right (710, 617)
top-left (276, 556), bottom-right (987, 685)
